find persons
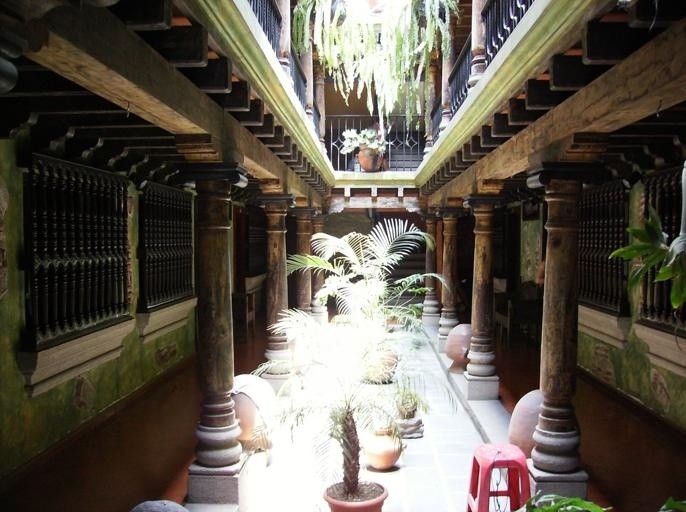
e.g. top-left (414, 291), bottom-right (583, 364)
top-left (372, 115), bottom-right (389, 171)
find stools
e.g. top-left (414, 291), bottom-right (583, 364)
top-left (467, 442), bottom-right (531, 512)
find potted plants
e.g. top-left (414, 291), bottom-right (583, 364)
top-left (340, 126), bottom-right (385, 171)
top-left (248, 217), bottom-right (446, 512)
